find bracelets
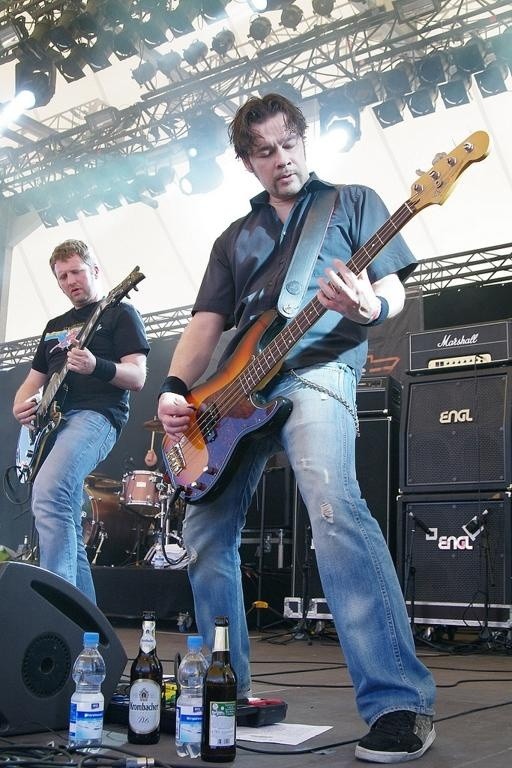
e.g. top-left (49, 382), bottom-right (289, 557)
top-left (360, 296), bottom-right (391, 328)
top-left (88, 356), bottom-right (117, 383)
top-left (158, 375), bottom-right (188, 399)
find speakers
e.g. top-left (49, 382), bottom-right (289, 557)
top-left (396, 492), bottom-right (512, 629)
top-left (293, 415), bottom-right (396, 620)
top-left (398, 367), bottom-right (512, 495)
top-left (0, 561), bottom-right (128, 737)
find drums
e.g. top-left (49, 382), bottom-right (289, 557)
top-left (118, 468), bottom-right (168, 517)
top-left (82, 472), bottom-right (148, 566)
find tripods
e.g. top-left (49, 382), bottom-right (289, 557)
top-left (404, 521), bottom-right (452, 653)
top-left (461, 526), bottom-right (512, 655)
top-left (246, 469), bottom-right (284, 626)
top-left (259, 520), bottom-right (340, 646)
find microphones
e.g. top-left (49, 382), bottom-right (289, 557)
top-left (466, 506), bottom-right (491, 533)
top-left (408, 511), bottom-right (432, 536)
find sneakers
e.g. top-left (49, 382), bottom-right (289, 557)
top-left (354, 709), bottom-right (438, 764)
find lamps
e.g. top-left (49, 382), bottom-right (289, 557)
top-left (12, 0), bottom-right (339, 125)
top-left (13, 103), bottom-right (234, 228)
top-left (308, 33), bottom-right (512, 153)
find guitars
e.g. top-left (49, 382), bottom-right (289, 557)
top-left (15, 265), bottom-right (145, 484)
top-left (162, 131), bottom-right (491, 506)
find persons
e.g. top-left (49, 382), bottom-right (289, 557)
top-left (11, 237), bottom-right (151, 620)
top-left (155, 90), bottom-right (439, 764)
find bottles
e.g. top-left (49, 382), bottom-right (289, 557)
top-left (200, 615), bottom-right (237, 763)
top-left (22, 535), bottom-right (31, 560)
top-left (154, 542), bottom-right (164, 569)
top-left (68, 632), bottom-right (106, 754)
top-left (127, 610), bottom-right (164, 745)
top-left (175, 635), bottom-right (210, 758)
